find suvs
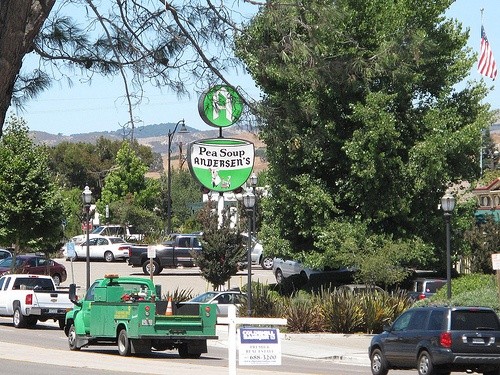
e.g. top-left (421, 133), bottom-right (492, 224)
top-left (368, 306), bottom-right (500, 375)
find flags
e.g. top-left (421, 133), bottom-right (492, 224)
top-left (477, 25), bottom-right (498, 81)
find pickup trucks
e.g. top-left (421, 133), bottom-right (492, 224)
top-left (64, 274), bottom-right (219, 358)
top-left (90, 224), bottom-right (272, 275)
top-left (0, 274), bottom-right (75, 329)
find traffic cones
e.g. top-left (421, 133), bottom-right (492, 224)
top-left (165, 297), bottom-right (172, 315)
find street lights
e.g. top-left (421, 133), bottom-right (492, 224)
top-left (491, 151), bottom-right (499, 169)
top-left (243, 188), bottom-right (257, 315)
top-left (248, 169), bottom-right (259, 239)
top-left (168, 118), bottom-right (189, 241)
top-left (82, 185), bottom-right (93, 297)
top-left (441, 195), bottom-right (454, 305)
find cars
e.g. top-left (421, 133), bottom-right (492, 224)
top-left (65, 237), bottom-right (132, 262)
top-left (273, 256), bottom-right (448, 328)
top-left (182, 291), bottom-right (248, 317)
top-left (0, 243), bottom-right (68, 288)
